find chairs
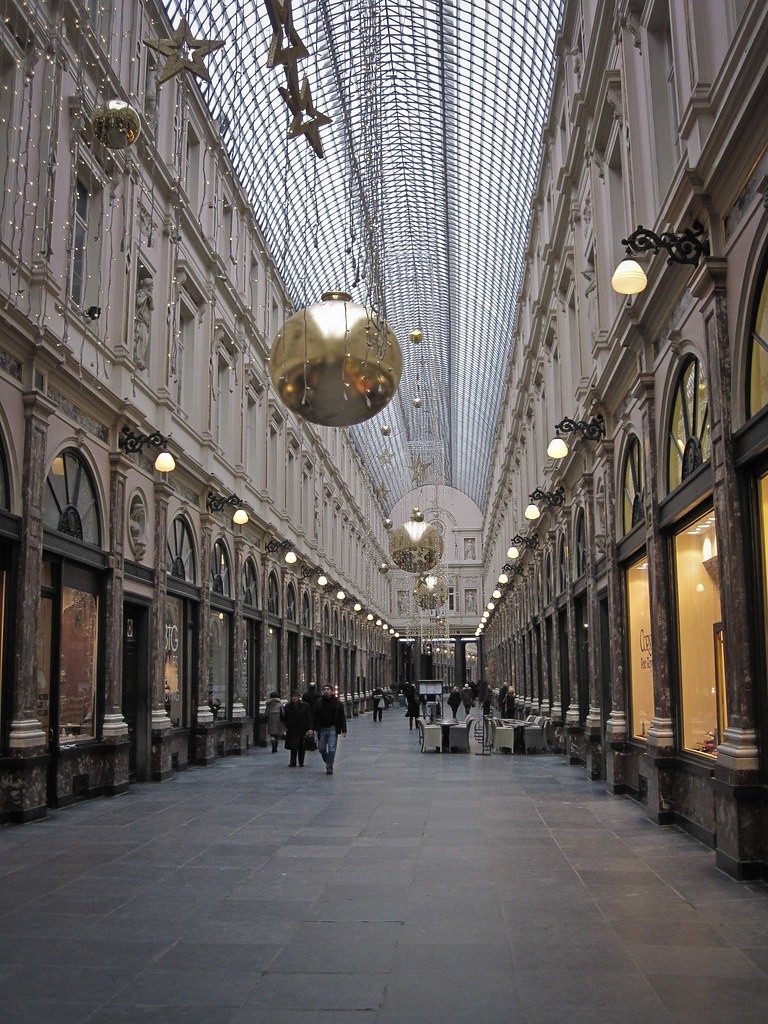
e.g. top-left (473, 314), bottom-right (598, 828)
top-left (492, 717), bottom-right (504, 727)
top-left (484, 714), bottom-right (493, 746)
top-left (416, 716), bottom-right (443, 753)
top-left (524, 715), bottom-right (537, 722)
top-left (521, 717), bottom-right (551, 756)
top-left (448, 717), bottom-right (475, 753)
top-left (459, 714), bottom-right (471, 724)
top-left (492, 718), bottom-right (514, 754)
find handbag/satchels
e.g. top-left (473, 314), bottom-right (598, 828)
top-left (303, 731), bottom-right (318, 751)
top-left (471, 702), bottom-right (476, 708)
top-left (378, 698), bottom-right (385, 709)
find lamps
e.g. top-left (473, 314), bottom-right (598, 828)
top-left (487, 594), bottom-right (506, 609)
top-left (391, 629), bottom-right (400, 638)
top-left (323, 583), bottom-right (346, 599)
top-left (357, 609), bottom-right (374, 622)
top-left (301, 566), bottom-right (327, 586)
top-left (374, 615), bottom-right (382, 626)
top-left (477, 628), bottom-right (485, 633)
top-left (265, 538), bottom-right (298, 564)
top-left (547, 414), bottom-right (605, 459)
top-left (524, 486), bottom-right (565, 520)
top-left (382, 620), bottom-right (388, 631)
top-left (492, 580), bottom-right (514, 599)
top-left (483, 610), bottom-right (495, 618)
top-left (342, 596), bottom-right (362, 612)
top-left (118, 426), bottom-right (176, 472)
top-left (609, 220), bottom-right (710, 294)
top-left (84, 306), bottom-right (102, 321)
top-left (475, 632), bottom-right (486, 637)
top-left (507, 533), bottom-right (539, 559)
top-left (479, 623), bottom-right (489, 629)
top-left (388, 624), bottom-right (395, 635)
top-left (207, 491), bottom-right (248, 525)
top-left (498, 563), bottom-right (524, 584)
top-left (480, 617), bottom-right (492, 623)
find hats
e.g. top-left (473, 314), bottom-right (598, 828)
top-left (270, 692), bottom-right (279, 699)
top-left (464, 683), bottom-right (469, 688)
top-left (324, 683), bottom-right (334, 691)
top-left (289, 690), bottom-right (301, 696)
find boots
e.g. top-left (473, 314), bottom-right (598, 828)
top-left (271, 740), bottom-right (279, 754)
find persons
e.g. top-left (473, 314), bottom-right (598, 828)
top-left (302, 681), bottom-right (321, 751)
top-left (466, 680), bottom-right (479, 700)
top-left (264, 692), bottom-right (284, 753)
top-left (462, 683), bottom-right (473, 715)
top-left (285, 689), bottom-right (313, 767)
top-left (307, 684), bottom-right (347, 775)
top-left (371, 685), bottom-right (394, 721)
top-left (478, 681), bottom-right (493, 716)
top-left (403, 680), bottom-right (441, 730)
top-left (497, 682), bottom-right (516, 719)
top-left (448, 686), bottom-right (461, 718)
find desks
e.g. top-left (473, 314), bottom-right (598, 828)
top-left (505, 721), bottom-right (533, 753)
top-left (432, 721), bottom-right (459, 748)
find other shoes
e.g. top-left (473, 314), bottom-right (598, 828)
top-left (327, 767), bottom-right (333, 775)
top-left (300, 764), bottom-right (304, 767)
top-left (288, 765), bottom-right (296, 767)
